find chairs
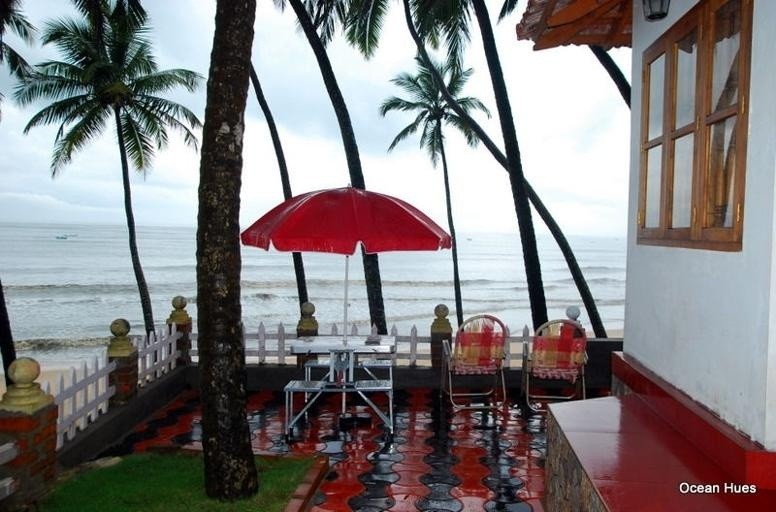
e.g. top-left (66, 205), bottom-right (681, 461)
top-left (520, 317), bottom-right (588, 415)
top-left (436, 312), bottom-right (510, 412)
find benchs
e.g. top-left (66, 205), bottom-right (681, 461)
top-left (282, 378), bottom-right (393, 445)
top-left (301, 357), bottom-right (396, 424)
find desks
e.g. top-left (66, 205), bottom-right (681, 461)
top-left (285, 333), bottom-right (398, 425)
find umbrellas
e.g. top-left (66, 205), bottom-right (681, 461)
top-left (239, 183), bottom-right (452, 432)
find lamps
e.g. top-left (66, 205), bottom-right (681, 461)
top-left (640, 1), bottom-right (672, 21)
